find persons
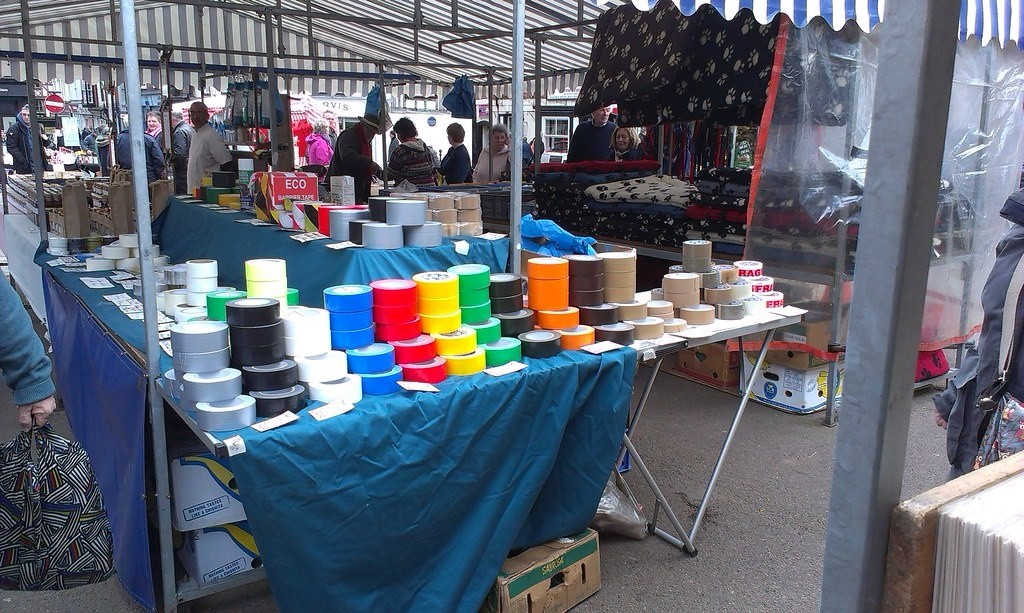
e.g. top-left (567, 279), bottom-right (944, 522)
top-left (80, 102), bottom-right (232, 212)
top-left (432, 123), bottom-right (473, 184)
top-left (932, 188), bottom-right (1024, 484)
top-left (324, 114), bottom-right (381, 205)
top-left (377, 118), bottom-right (437, 191)
top-left (473, 125), bottom-right (544, 184)
top-left (0, 266), bottom-right (57, 433)
top-left (308, 119), bottom-right (333, 167)
top-left (0, 104), bottom-right (64, 175)
top-left (566, 105), bottom-right (647, 162)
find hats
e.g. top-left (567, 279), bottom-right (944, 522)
top-left (357, 113), bottom-right (383, 135)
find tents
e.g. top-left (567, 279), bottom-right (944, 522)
top-left (169, 93), bottom-right (338, 172)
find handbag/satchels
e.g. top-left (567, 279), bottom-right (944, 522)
top-left (0, 418), bottom-right (116, 592)
top-left (972, 394), bottom-right (1024, 468)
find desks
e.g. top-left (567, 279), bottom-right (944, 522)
top-left (46, 164), bottom-right (100, 173)
top-left (0, 194), bottom-right (852, 613)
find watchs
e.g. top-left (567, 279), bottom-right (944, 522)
top-left (976, 379), bottom-right (1007, 412)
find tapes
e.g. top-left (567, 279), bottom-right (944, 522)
top-left (133, 264), bottom-right (187, 297)
top-left (166, 259), bottom-right (362, 432)
top-left (323, 264), bottom-right (522, 351)
top-left (524, 301), bottom-right (665, 350)
top-left (191, 171), bottom-right (241, 210)
top-left (527, 254), bottom-right (635, 310)
top-left (85, 234), bottom-right (169, 272)
top-left (271, 198), bottom-right (369, 240)
top-left (347, 308), bottom-right (562, 396)
top-left (647, 301), bottom-right (687, 332)
top-left (349, 196), bottom-right (442, 249)
top-left (394, 192), bottom-right (483, 236)
top-left (667, 260), bottom-right (786, 324)
top-left (682, 240), bottom-right (713, 272)
top-left (48, 235), bottom-right (119, 255)
top-left (653, 273), bottom-right (715, 323)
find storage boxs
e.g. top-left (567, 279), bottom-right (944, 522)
top-left (749, 300), bottom-right (849, 372)
top-left (480, 527), bottom-right (600, 613)
top-left (738, 352), bottom-right (844, 414)
top-left (268, 171), bottom-right (318, 210)
top-left (168, 442), bottom-right (247, 532)
top-left (172, 522), bottom-right (263, 586)
top-left (330, 176), bottom-right (355, 205)
top-left (678, 343), bottom-right (741, 387)
